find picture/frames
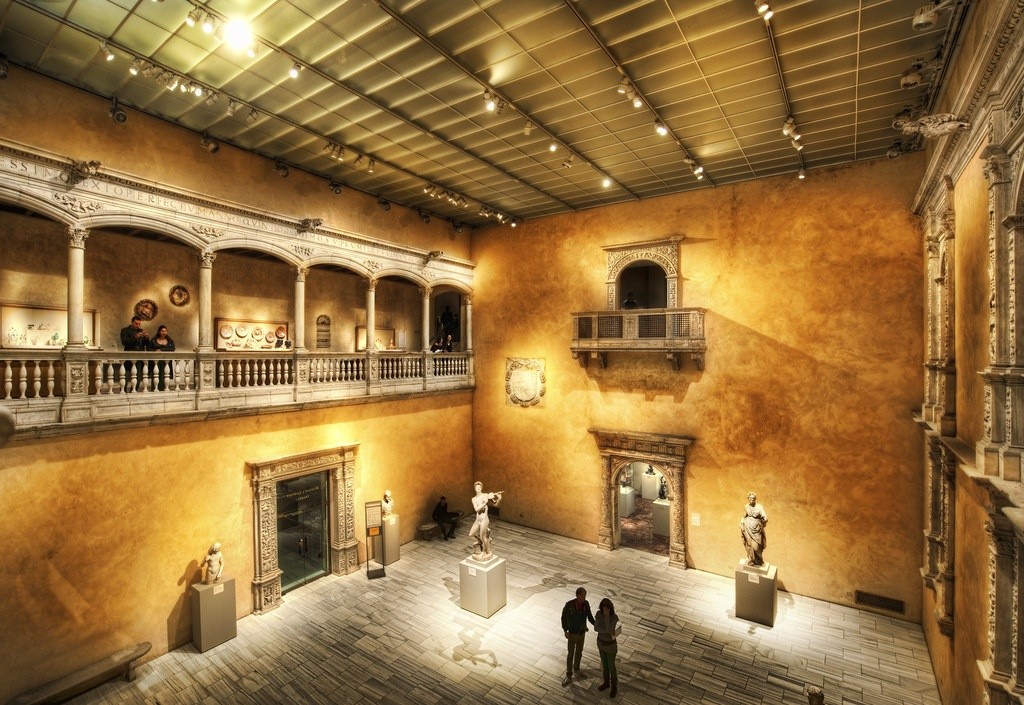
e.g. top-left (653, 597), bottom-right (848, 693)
top-left (356, 326), bottom-right (396, 352)
top-left (0, 299), bottom-right (104, 350)
top-left (214, 318), bottom-right (289, 352)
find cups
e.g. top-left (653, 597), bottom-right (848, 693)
top-left (277, 339), bottom-right (283, 346)
top-left (285, 340), bottom-right (291, 347)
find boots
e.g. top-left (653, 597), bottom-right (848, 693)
top-left (599, 678), bottom-right (610, 691)
top-left (610, 678), bottom-right (620, 698)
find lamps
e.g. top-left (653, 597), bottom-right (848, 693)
top-left (562, 151), bottom-right (574, 169)
top-left (753, 0), bottom-right (769, 13)
top-left (98, 43), bottom-right (259, 124)
top-left (790, 132), bottom-right (801, 140)
top-left (759, 10), bottom-right (774, 21)
top-left (690, 165), bottom-right (704, 174)
top-left (791, 140), bottom-right (803, 151)
top-left (695, 173), bottom-right (703, 180)
top-left (799, 168), bottom-right (806, 179)
top-left (322, 141), bottom-right (375, 174)
top-left (483, 90), bottom-right (533, 136)
top-left (185, 6), bottom-right (214, 35)
top-left (632, 95), bottom-right (642, 108)
top-left (626, 85), bottom-right (635, 101)
top-left (424, 185), bottom-right (517, 229)
top-left (654, 117), bottom-right (667, 136)
top-left (644, 465), bottom-right (655, 477)
top-left (215, 20), bottom-right (229, 42)
top-left (290, 64), bottom-right (302, 79)
top-left (782, 115), bottom-right (796, 135)
top-left (618, 77), bottom-right (628, 93)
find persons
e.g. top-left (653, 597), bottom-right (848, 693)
top-left (594, 598), bottom-right (622, 698)
top-left (432, 496), bottom-right (457, 540)
top-left (121, 316), bottom-right (149, 386)
top-left (431, 337), bottom-right (443, 352)
top-left (623, 292), bottom-right (638, 308)
top-left (444, 334), bottom-right (454, 352)
top-left (561, 587), bottom-right (595, 687)
top-left (468, 481), bottom-right (505, 561)
top-left (147, 325), bottom-right (175, 386)
top-left (739, 492), bottom-right (769, 566)
top-left (200, 542), bottom-right (225, 583)
top-left (382, 490), bottom-right (394, 518)
top-left (807, 686), bottom-right (824, 705)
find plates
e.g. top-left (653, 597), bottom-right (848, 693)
top-left (236, 324), bottom-right (248, 337)
top-left (220, 325), bottom-right (233, 338)
top-left (251, 328), bottom-right (264, 341)
top-left (246, 338), bottom-right (258, 348)
top-left (266, 331), bottom-right (275, 343)
top-left (276, 326), bottom-right (286, 338)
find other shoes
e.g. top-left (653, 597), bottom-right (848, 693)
top-left (444, 534), bottom-right (448, 540)
top-left (562, 676), bottom-right (571, 687)
top-left (448, 533), bottom-right (456, 538)
top-left (575, 671), bottom-right (587, 678)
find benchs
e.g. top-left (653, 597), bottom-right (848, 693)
top-left (416, 509), bottom-right (464, 541)
top-left (0, 641), bottom-right (152, 705)
